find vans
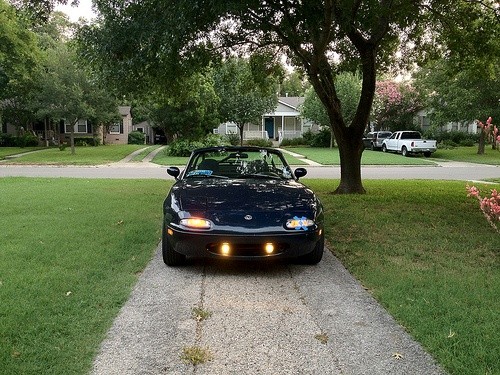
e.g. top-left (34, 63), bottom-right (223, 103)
top-left (366, 131), bottom-right (391, 151)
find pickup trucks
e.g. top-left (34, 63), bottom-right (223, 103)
top-left (382, 130), bottom-right (437, 157)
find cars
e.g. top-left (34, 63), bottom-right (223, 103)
top-left (160, 146), bottom-right (326, 267)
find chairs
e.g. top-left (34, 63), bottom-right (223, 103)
top-left (198, 158), bottom-right (220, 172)
top-left (247, 159), bottom-right (270, 175)
